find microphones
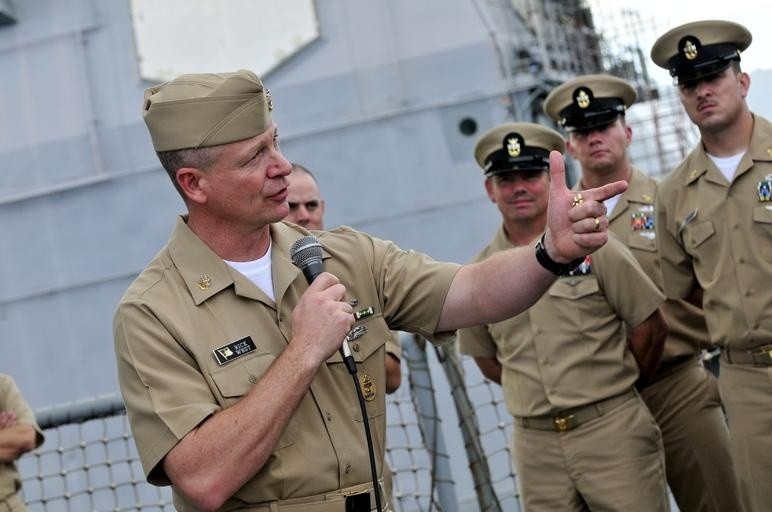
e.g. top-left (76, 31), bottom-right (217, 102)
top-left (290, 237), bottom-right (358, 374)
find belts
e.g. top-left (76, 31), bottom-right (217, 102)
top-left (513, 387), bottom-right (639, 432)
top-left (722, 344), bottom-right (772, 366)
top-left (226, 479), bottom-right (388, 512)
top-left (636, 338), bottom-right (700, 392)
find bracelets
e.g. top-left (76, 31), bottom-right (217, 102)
top-left (534, 232), bottom-right (587, 276)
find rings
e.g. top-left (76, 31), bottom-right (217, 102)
top-left (593, 217), bottom-right (602, 229)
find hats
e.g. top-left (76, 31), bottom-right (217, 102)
top-left (474, 75), bottom-right (637, 176)
top-left (651, 20), bottom-right (752, 85)
top-left (143, 69), bottom-right (272, 151)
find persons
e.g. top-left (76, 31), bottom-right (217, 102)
top-left (541, 73), bottom-right (743, 512)
top-left (649, 18), bottom-right (772, 510)
top-left (455, 119), bottom-right (670, 512)
top-left (111, 67), bottom-right (634, 512)
top-left (0, 371), bottom-right (45, 512)
top-left (279, 161), bottom-right (404, 396)
